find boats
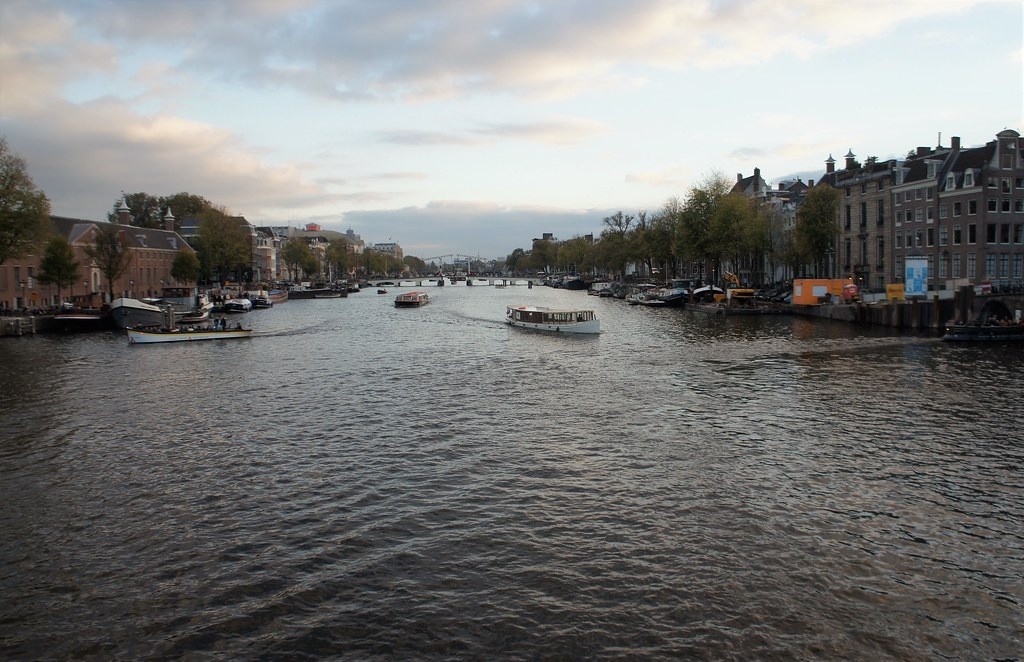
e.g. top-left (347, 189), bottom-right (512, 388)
top-left (224, 299), bottom-right (252, 313)
top-left (181, 312), bottom-right (209, 323)
top-left (505, 303), bottom-right (601, 334)
top-left (126, 327), bottom-right (253, 344)
top-left (377, 289), bottom-right (387, 294)
top-left (393, 291), bottom-right (429, 308)
top-left (625, 279), bottom-right (695, 306)
top-left (942, 324), bottom-right (1024, 341)
top-left (53, 301), bottom-right (112, 332)
top-left (251, 296), bottom-right (273, 309)
top-left (109, 287), bottom-right (214, 328)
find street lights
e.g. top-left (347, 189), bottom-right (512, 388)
top-left (130, 279), bottom-right (135, 298)
top-left (19, 279), bottom-right (26, 307)
top-left (83, 278), bottom-right (88, 302)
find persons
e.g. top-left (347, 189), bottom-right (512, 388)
top-left (133, 324), bottom-right (212, 333)
top-left (955, 315), bottom-right (1024, 326)
top-left (577, 314), bottom-right (582, 322)
top-left (239, 291), bottom-right (249, 299)
top-left (990, 286), bottom-right (1024, 294)
top-left (235, 323), bottom-right (242, 330)
top-left (213, 293), bottom-right (226, 306)
top-left (214, 315), bottom-right (226, 331)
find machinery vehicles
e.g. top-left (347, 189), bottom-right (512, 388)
top-left (714, 271), bottom-right (757, 309)
